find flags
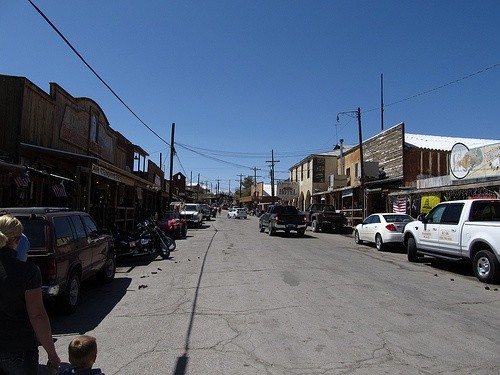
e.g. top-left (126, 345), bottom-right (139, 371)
top-left (420, 192), bottom-right (442, 213)
top-left (13, 172), bottom-right (31, 187)
top-left (51, 182), bottom-right (67, 198)
top-left (392, 197), bottom-right (406, 214)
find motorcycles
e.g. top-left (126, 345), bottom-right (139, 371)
top-left (104, 212), bottom-right (176, 260)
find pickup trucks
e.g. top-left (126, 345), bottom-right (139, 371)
top-left (402, 197), bottom-right (500, 282)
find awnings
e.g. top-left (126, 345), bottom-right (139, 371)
top-left (21, 143), bottom-right (162, 191)
top-left (312, 177), bottom-right (401, 198)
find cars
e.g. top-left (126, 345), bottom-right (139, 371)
top-left (353, 212), bottom-right (416, 251)
top-left (152, 212), bottom-right (187, 239)
top-left (259, 205), bottom-right (309, 236)
top-left (227, 208), bottom-right (248, 219)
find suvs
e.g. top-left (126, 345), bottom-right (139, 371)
top-left (303, 203), bottom-right (344, 233)
top-left (180, 203), bottom-right (204, 226)
top-left (0, 206), bottom-right (117, 306)
top-left (199, 204), bottom-right (211, 221)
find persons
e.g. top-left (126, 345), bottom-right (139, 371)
top-left (47, 335), bottom-right (105, 375)
top-left (0, 214), bottom-right (61, 375)
